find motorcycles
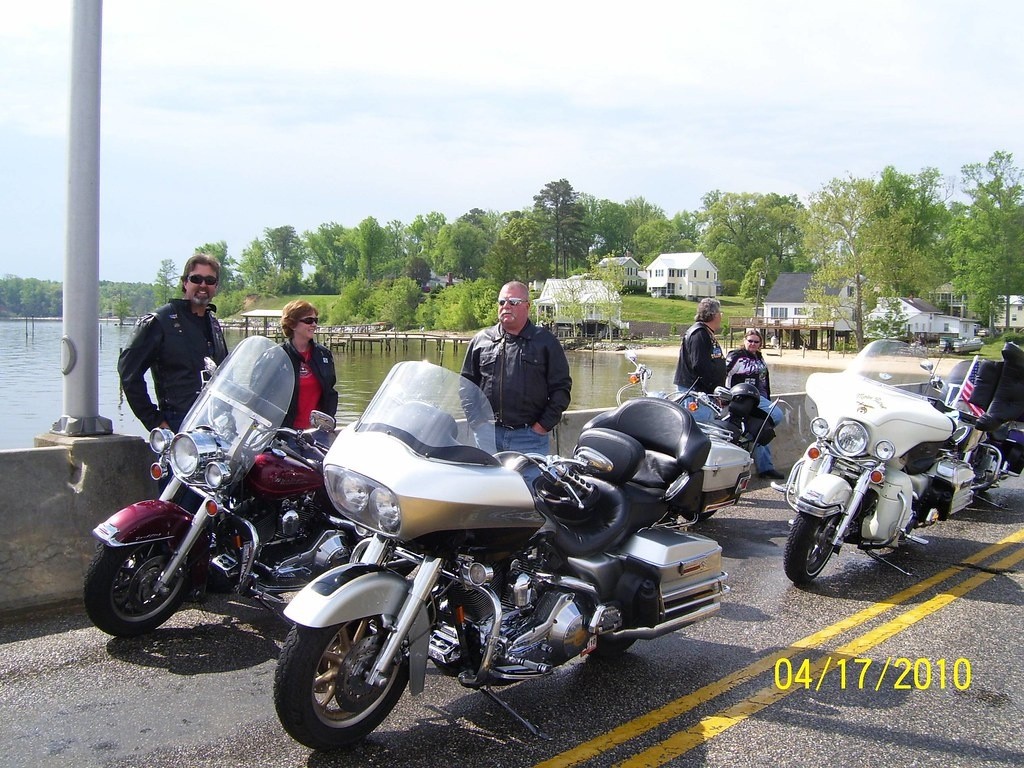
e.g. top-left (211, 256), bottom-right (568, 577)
top-left (272, 361), bottom-right (731, 754)
top-left (83, 336), bottom-right (462, 637)
top-left (615, 343), bottom-right (755, 525)
top-left (770, 338), bottom-right (1024, 585)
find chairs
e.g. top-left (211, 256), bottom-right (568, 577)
top-left (939, 360), bottom-right (973, 404)
top-left (580, 397), bottom-right (712, 528)
top-left (520, 427), bottom-right (645, 555)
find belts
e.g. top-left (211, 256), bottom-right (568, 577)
top-left (496, 422), bottom-right (535, 430)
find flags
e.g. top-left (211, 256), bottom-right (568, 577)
top-left (959, 355), bottom-right (987, 417)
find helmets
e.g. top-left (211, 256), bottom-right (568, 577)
top-left (532, 475), bottom-right (599, 521)
top-left (729, 383), bottom-right (760, 409)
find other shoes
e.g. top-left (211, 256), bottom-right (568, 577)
top-left (759, 470), bottom-right (784, 479)
top-left (207, 581), bottom-right (233, 593)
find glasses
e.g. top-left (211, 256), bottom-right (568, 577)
top-left (188, 274), bottom-right (218, 285)
top-left (300, 317), bottom-right (318, 324)
top-left (499, 299), bottom-right (527, 306)
top-left (748, 339), bottom-right (760, 343)
top-left (718, 311), bottom-right (724, 316)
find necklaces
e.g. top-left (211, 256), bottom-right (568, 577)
top-left (300, 345), bottom-right (310, 371)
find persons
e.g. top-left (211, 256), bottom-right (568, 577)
top-left (673, 296), bottom-right (728, 425)
top-left (725, 331), bottom-right (788, 481)
top-left (458, 281), bottom-right (572, 456)
top-left (250, 299), bottom-right (339, 462)
top-left (118, 253), bottom-right (236, 602)
